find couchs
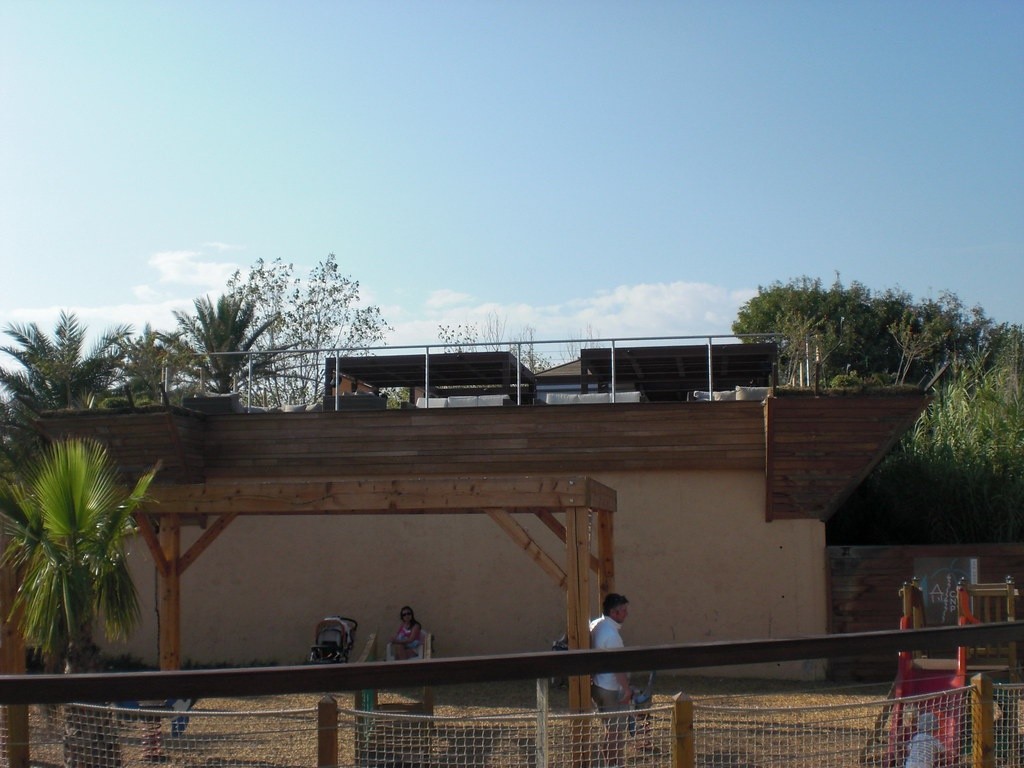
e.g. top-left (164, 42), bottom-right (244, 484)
top-left (184, 391), bottom-right (388, 412)
top-left (401, 395), bottom-right (512, 408)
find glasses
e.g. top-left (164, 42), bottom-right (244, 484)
top-left (402, 612), bottom-right (409, 616)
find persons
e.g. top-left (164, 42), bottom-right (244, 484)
top-left (905, 713), bottom-right (952, 768)
top-left (589, 593), bottom-right (633, 768)
top-left (389, 605), bottom-right (422, 660)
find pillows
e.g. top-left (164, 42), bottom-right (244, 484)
top-left (712, 385), bottom-right (769, 401)
top-left (284, 404), bottom-right (321, 413)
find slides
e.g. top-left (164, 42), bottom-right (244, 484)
top-left (885, 612), bottom-right (967, 767)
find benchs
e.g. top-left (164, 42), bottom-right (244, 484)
top-left (532, 392), bottom-right (649, 405)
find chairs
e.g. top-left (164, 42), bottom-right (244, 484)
top-left (385, 630), bottom-right (432, 661)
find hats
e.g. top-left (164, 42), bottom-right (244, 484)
top-left (602, 593), bottom-right (629, 608)
top-left (917, 714), bottom-right (940, 730)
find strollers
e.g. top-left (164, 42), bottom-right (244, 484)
top-left (306, 615), bottom-right (359, 665)
